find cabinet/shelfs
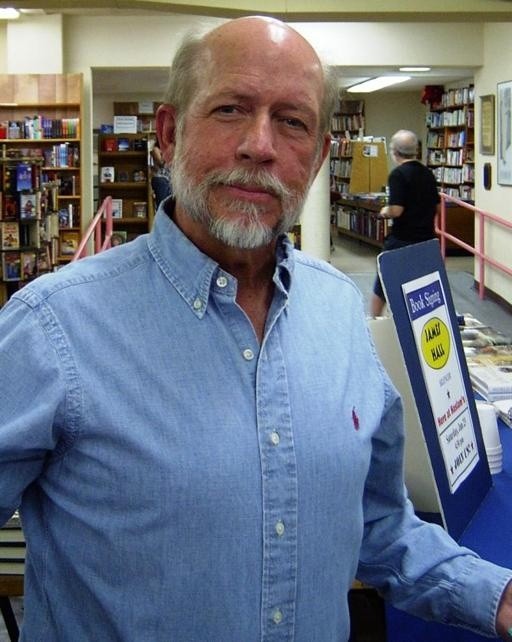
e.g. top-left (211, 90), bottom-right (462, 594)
top-left (0, 73), bottom-right (84, 306)
top-left (98, 102), bottom-right (164, 247)
top-left (327, 83), bottom-right (477, 256)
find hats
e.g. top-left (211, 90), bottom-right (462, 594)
top-left (389, 130), bottom-right (418, 155)
